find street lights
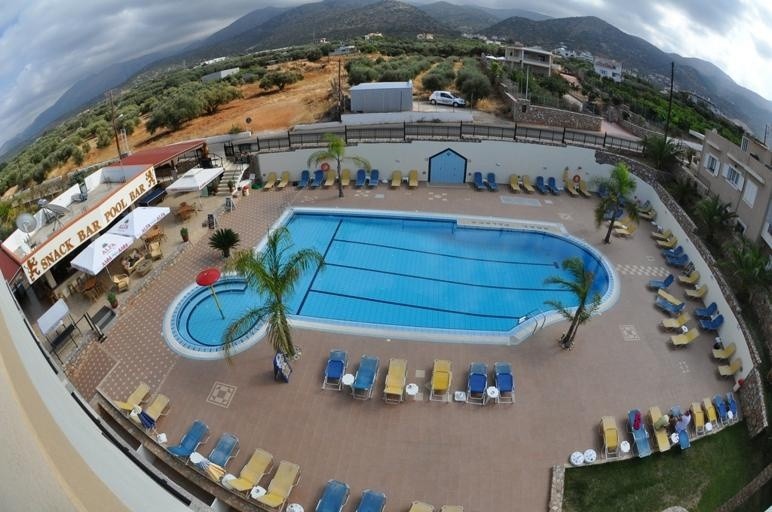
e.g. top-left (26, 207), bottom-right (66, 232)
top-left (112, 112), bottom-right (123, 161)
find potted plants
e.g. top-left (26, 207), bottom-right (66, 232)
top-left (227, 180), bottom-right (235, 193)
top-left (212, 180), bottom-right (219, 193)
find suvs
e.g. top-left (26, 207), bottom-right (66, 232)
top-left (428, 90), bottom-right (465, 109)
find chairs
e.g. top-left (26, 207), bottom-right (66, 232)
top-left (471, 166), bottom-right (591, 200)
top-left (50, 201), bottom-right (201, 311)
top-left (595, 391), bottom-right (744, 462)
top-left (600, 184), bottom-right (743, 378)
top-left (109, 349), bottom-right (521, 512)
top-left (261, 167), bottom-right (422, 192)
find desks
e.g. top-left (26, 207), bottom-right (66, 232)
top-left (584, 448), bottom-right (596, 462)
top-left (139, 189), bottom-right (168, 207)
top-left (569, 449), bottom-right (584, 465)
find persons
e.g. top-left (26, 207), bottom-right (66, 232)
top-left (654, 414), bottom-right (673, 431)
top-left (672, 409), bottom-right (691, 433)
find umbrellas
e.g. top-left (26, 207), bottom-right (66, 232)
top-left (165, 168), bottom-right (224, 208)
top-left (70, 233), bottom-right (134, 288)
top-left (106, 206), bottom-right (171, 250)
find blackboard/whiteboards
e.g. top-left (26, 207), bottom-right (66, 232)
top-left (208, 214), bottom-right (215, 229)
top-left (226, 197), bottom-right (232, 212)
